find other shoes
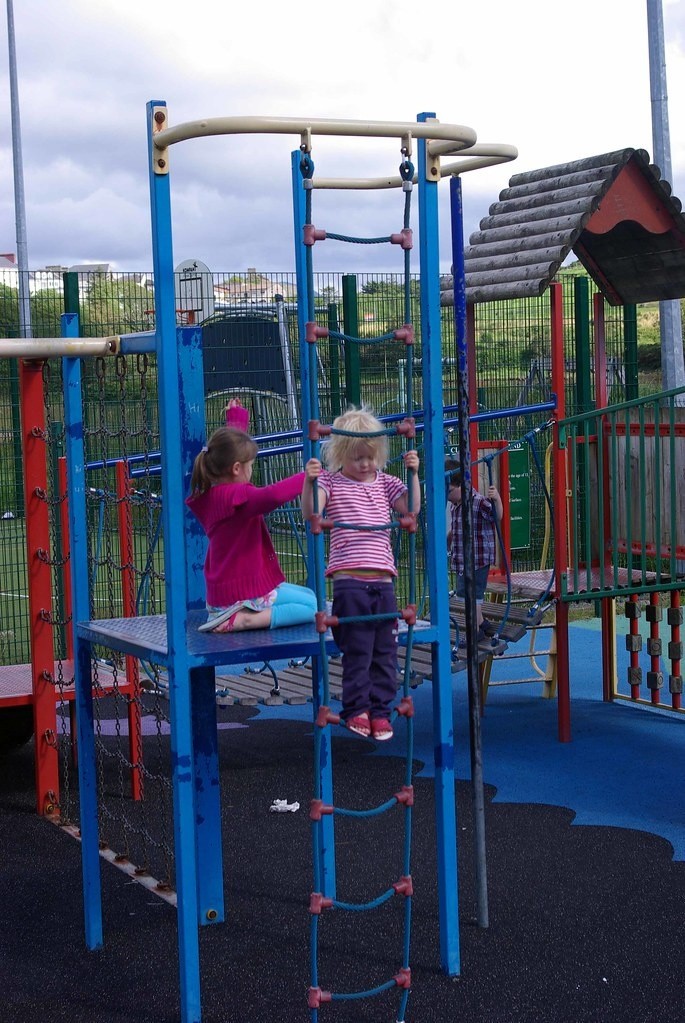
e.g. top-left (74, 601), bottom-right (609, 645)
top-left (455, 618), bottom-right (496, 651)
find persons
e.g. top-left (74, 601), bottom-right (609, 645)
top-left (302, 400), bottom-right (421, 739)
top-left (440, 458), bottom-right (504, 649)
top-left (185, 396), bottom-right (320, 632)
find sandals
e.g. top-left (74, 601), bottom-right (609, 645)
top-left (372, 717), bottom-right (394, 741)
top-left (346, 709), bottom-right (371, 737)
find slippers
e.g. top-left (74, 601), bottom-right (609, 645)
top-left (197, 602), bottom-right (247, 632)
